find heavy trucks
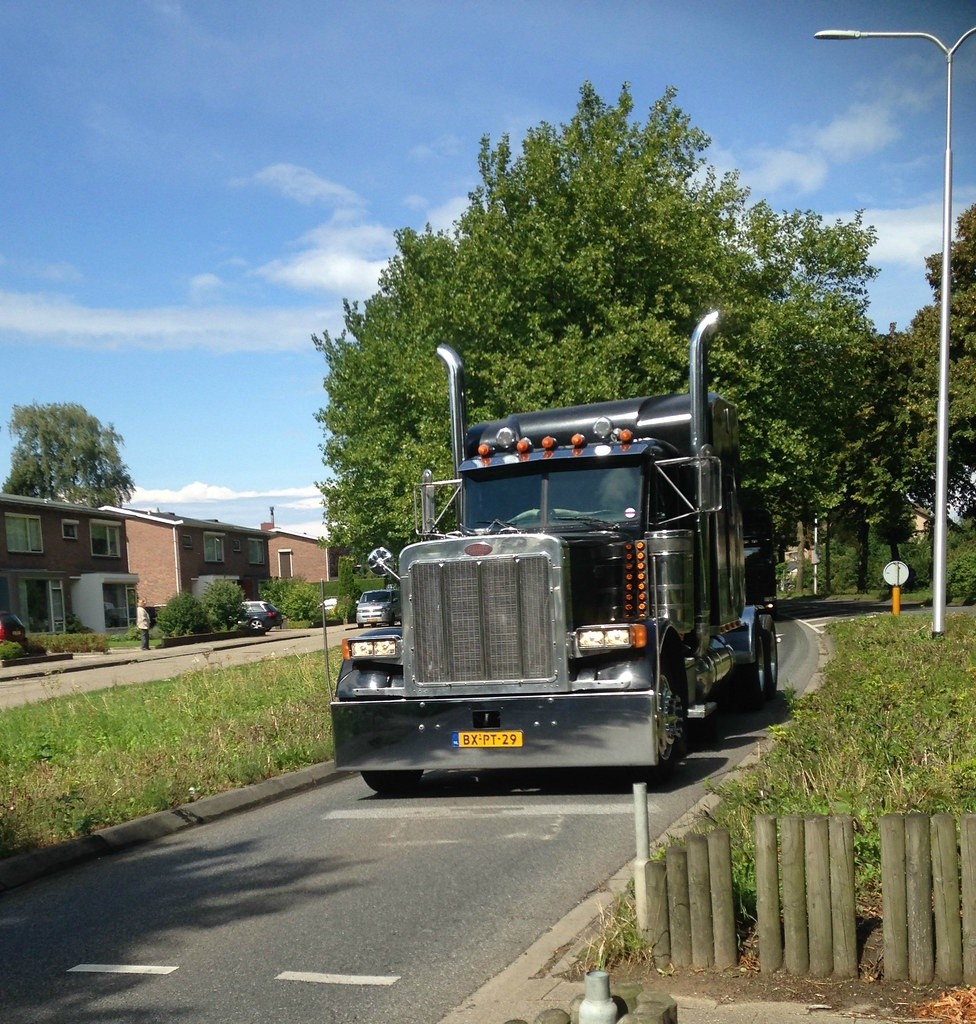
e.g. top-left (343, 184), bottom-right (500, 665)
top-left (329, 309), bottom-right (785, 793)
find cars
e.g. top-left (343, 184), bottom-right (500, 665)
top-left (0, 611), bottom-right (29, 651)
top-left (321, 598), bottom-right (338, 609)
top-left (144, 606), bottom-right (161, 627)
top-left (779, 578), bottom-right (796, 593)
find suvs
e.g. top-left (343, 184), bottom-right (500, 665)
top-left (238, 601), bottom-right (283, 632)
top-left (106, 606), bottom-right (136, 628)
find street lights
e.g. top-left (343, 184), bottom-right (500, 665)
top-left (817, 27), bottom-right (976, 640)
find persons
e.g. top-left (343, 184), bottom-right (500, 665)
top-left (137, 600), bottom-right (151, 650)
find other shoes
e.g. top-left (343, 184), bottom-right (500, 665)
top-left (142, 647), bottom-right (151, 650)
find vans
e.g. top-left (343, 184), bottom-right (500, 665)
top-left (356, 589), bottom-right (402, 628)
